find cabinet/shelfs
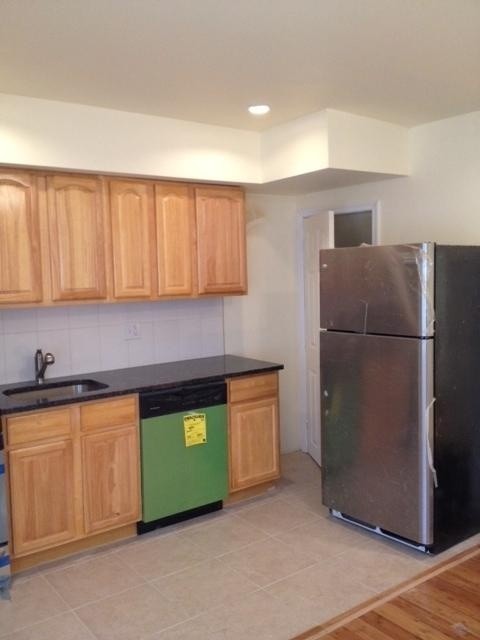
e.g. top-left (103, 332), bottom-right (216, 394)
top-left (226, 370), bottom-right (282, 495)
top-left (2, 393), bottom-right (144, 561)
top-left (0, 165), bottom-right (249, 309)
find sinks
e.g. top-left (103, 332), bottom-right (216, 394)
top-left (3, 379), bottom-right (108, 402)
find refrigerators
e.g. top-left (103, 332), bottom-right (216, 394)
top-left (319, 240), bottom-right (480, 556)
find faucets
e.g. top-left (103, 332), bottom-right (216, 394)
top-left (34, 347), bottom-right (54, 382)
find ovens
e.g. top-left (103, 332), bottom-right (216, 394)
top-left (139, 382), bottom-right (229, 536)
